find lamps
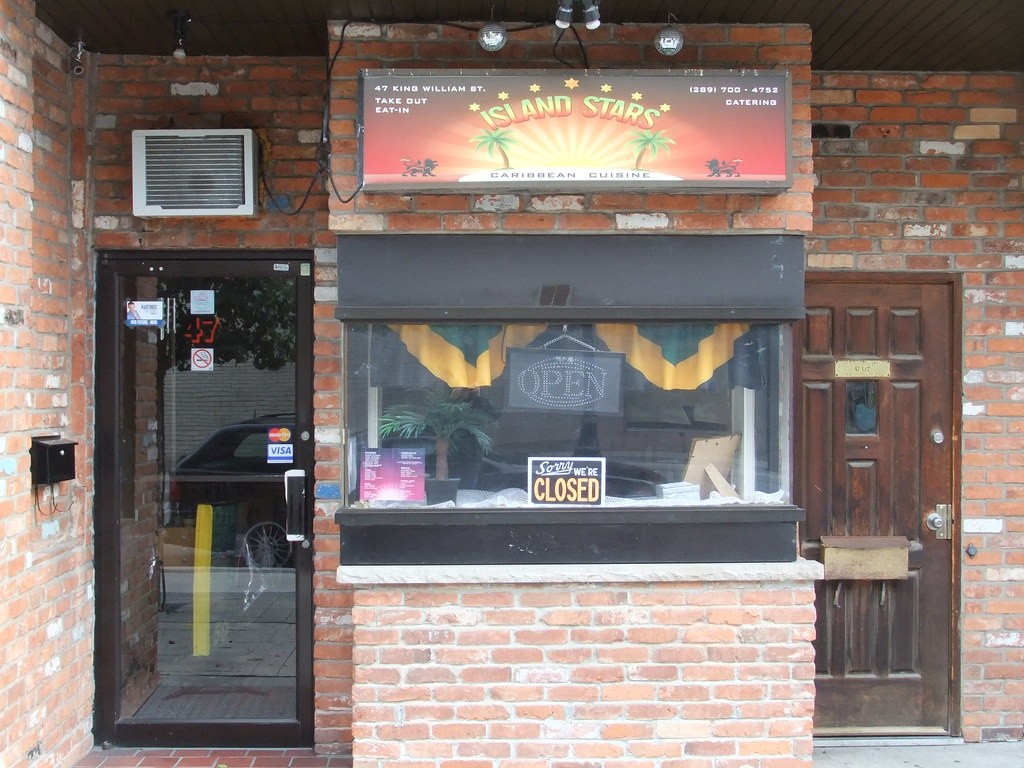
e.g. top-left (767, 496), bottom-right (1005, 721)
top-left (166, 9), bottom-right (192, 59)
top-left (581, 0), bottom-right (601, 30)
top-left (555, 0), bottom-right (574, 29)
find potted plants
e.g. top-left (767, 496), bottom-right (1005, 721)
top-left (377, 386), bottom-right (501, 505)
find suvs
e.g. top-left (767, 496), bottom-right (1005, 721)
top-left (158, 412), bottom-right (298, 569)
